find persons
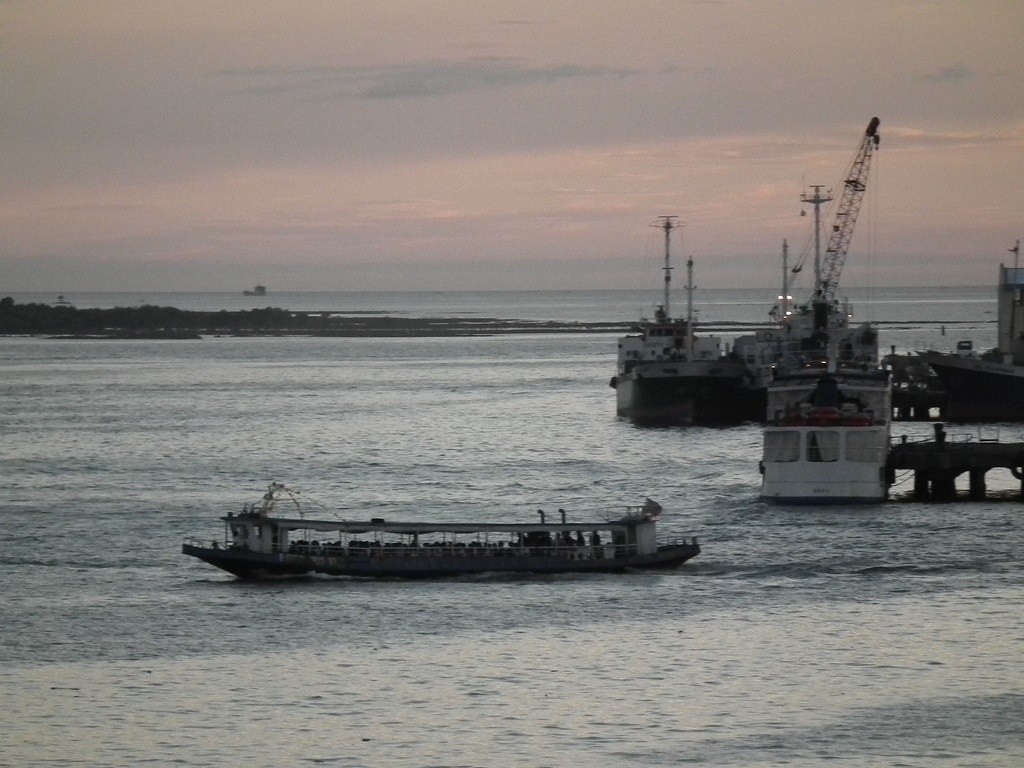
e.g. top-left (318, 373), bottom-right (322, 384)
top-left (670, 351), bottom-right (677, 360)
top-left (589, 530), bottom-right (601, 546)
top-left (539, 532), bottom-right (551, 547)
top-left (517, 533), bottom-right (530, 547)
top-left (233, 531), bottom-right (239, 545)
top-left (553, 531), bottom-right (585, 546)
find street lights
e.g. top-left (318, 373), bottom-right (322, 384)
top-left (1007, 247), bottom-right (1019, 353)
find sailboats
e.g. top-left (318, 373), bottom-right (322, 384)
top-left (731, 239), bottom-right (878, 406)
top-left (614, 216), bottom-right (741, 422)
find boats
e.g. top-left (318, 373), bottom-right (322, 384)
top-left (241, 285), bottom-right (265, 297)
top-left (914, 339), bottom-right (980, 359)
top-left (183, 484), bottom-right (700, 579)
top-left (760, 184), bottom-right (893, 507)
